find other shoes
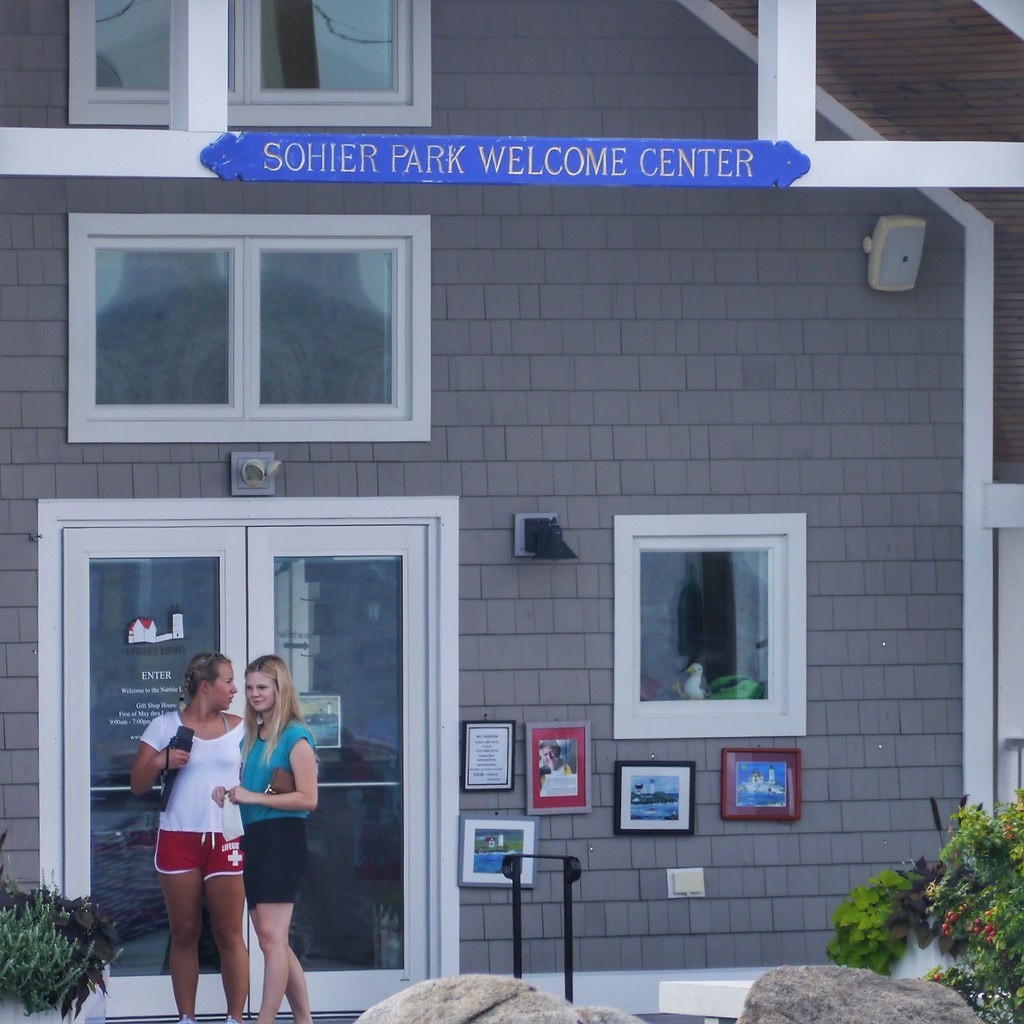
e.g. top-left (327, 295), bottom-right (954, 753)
top-left (177, 1014), bottom-right (197, 1024)
top-left (225, 1015), bottom-right (244, 1024)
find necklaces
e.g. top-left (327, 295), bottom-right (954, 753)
top-left (187, 710), bottom-right (217, 727)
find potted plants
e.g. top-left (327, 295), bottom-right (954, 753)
top-left (0, 868), bottom-right (127, 1024)
top-left (828, 863), bottom-right (977, 980)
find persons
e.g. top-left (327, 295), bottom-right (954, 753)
top-left (211, 654), bottom-right (319, 1024)
top-left (539, 740), bottom-right (572, 790)
top-left (131, 652), bottom-right (249, 1024)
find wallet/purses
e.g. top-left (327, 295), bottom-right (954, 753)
top-left (264, 767), bottom-right (296, 793)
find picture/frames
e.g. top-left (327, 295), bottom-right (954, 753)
top-left (456, 815), bottom-right (542, 890)
top-left (719, 747), bottom-right (803, 821)
top-left (527, 720), bottom-right (593, 816)
top-left (461, 720), bottom-right (517, 792)
top-left (610, 761), bottom-right (698, 835)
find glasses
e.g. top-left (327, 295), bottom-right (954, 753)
top-left (201, 651), bottom-right (224, 672)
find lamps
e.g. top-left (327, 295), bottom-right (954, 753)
top-left (513, 512), bottom-right (578, 564)
top-left (862, 214), bottom-right (926, 290)
top-left (230, 452), bottom-right (283, 496)
